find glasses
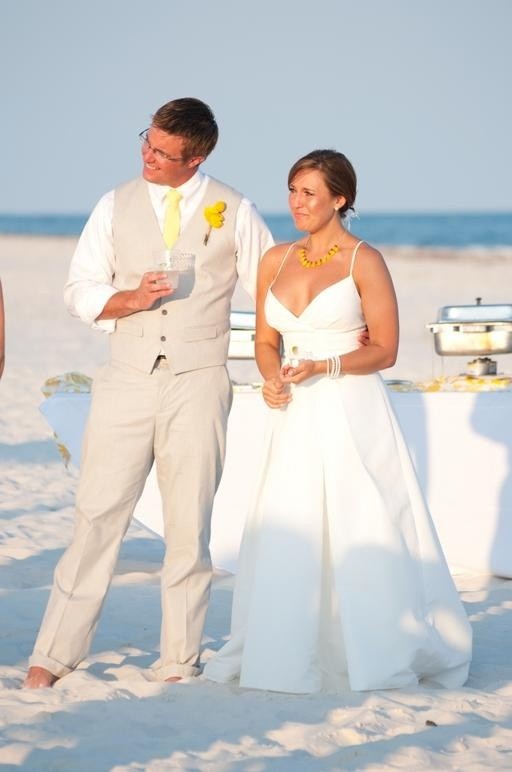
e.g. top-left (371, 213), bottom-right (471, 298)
top-left (138, 128), bottom-right (190, 164)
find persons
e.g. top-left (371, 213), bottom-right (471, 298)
top-left (205, 151), bottom-right (475, 691)
top-left (21, 98), bottom-right (370, 689)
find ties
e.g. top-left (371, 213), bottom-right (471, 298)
top-left (163, 189), bottom-right (183, 250)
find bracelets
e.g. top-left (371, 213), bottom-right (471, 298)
top-left (326, 357), bottom-right (341, 377)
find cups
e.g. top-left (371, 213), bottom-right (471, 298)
top-left (151, 248), bottom-right (179, 290)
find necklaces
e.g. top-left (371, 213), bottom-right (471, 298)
top-left (298, 228), bottom-right (348, 267)
